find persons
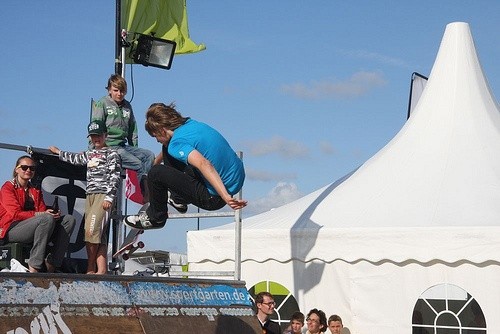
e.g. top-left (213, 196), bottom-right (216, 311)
top-left (48, 120), bottom-right (123, 275)
top-left (250, 292), bottom-right (282, 334)
top-left (91, 74), bottom-right (155, 214)
top-left (123, 103), bottom-right (248, 230)
top-left (282, 312), bottom-right (305, 334)
top-left (0, 155), bottom-right (76, 273)
top-left (306, 308), bottom-right (328, 334)
top-left (328, 314), bottom-right (343, 334)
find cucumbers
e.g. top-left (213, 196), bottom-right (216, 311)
top-left (112, 201), bottom-right (151, 260)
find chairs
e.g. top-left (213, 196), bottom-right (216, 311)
top-left (168, 190), bottom-right (189, 214)
top-left (123, 210), bottom-right (167, 230)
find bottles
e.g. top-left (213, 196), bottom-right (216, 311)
top-left (87, 119), bottom-right (107, 138)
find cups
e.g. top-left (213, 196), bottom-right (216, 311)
top-left (306, 317), bottom-right (319, 324)
top-left (261, 302), bottom-right (276, 307)
top-left (18, 165), bottom-right (36, 171)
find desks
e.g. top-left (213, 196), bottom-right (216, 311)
top-left (111, 0), bottom-right (176, 276)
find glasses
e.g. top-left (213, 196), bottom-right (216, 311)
top-left (116, 33), bottom-right (177, 71)
